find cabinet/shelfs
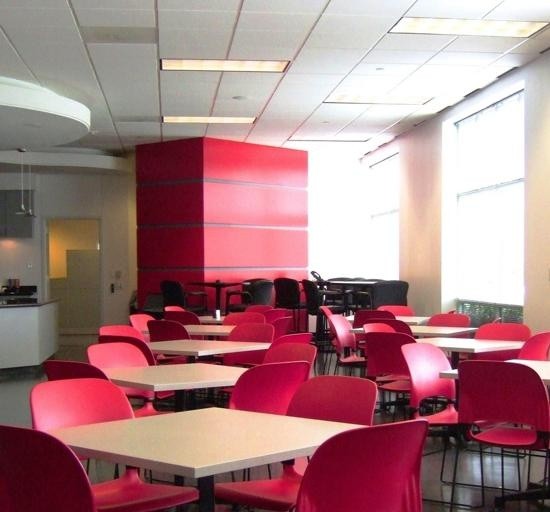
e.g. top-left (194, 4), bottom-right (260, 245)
top-left (0, 297), bottom-right (64, 375)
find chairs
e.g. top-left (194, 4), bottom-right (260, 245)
top-left (225, 279), bottom-right (274, 313)
top-left (268, 316), bottom-right (295, 338)
top-left (160, 279), bottom-right (206, 317)
top-left (98, 335), bottom-right (176, 401)
top-left (302, 279), bottom-right (339, 341)
top-left (329, 313), bottom-right (366, 376)
top-left (371, 280), bottom-right (409, 310)
top-left (361, 323), bottom-right (403, 413)
top-left (518, 331), bottom-right (549, 360)
top-left (426, 314), bottom-right (472, 327)
top-left (223, 312), bottom-right (264, 327)
top-left (365, 332), bottom-right (417, 422)
top-left (264, 309), bottom-right (288, 320)
top-left (234, 332), bottom-right (313, 368)
top-left (0, 425), bottom-right (199, 511)
top-left (294, 418), bottom-right (430, 512)
top-left (99, 325), bottom-right (149, 345)
top-left (274, 278), bottom-right (308, 333)
top-left (164, 311), bottom-right (201, 326)
top-left (244, 304), bottom-right (273, 315)
top-left (223, 323), bottom-right (274, 366)
top-left (376, 305), bottom-right (416, 317)
top-left (320, 305), bottom-right (348, 375)
top-left (43, 359), bottom-right (176, 482)
top-left (30, 378), bottom-right (135, 477)
top-left (148, 320), bottom-right (191, 341)
top-left (352, 310), bottom-right (395, 328)
top-left (129, 314), bottom-right (183, 359)
top-left (402, 342), bottom-right (505, 510)
top-left (163, 305), bottom-right (190, 316)
top-left (474, 322), bottom-right (530, 340)
top-left (283, 375), bottom-right (378, 427)
top-left (262, 343), bottom-right (317, 371)
top-left (87, 342), bottom-right (176, 403)
top-left (227, 360), bottom-right (311, 481)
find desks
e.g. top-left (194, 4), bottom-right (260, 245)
top-left (45, 406), bottom-right (372, 512)
top-left (144, 339), bottom-right (272, 409)
top-left (198, 314), bottom-right (228, 360)
top-left (184, 324), bottom-right (237, 395)
top-left (324, 279), bottom-right (381, 309)
top-left (102, 362), bottom-right (251, 512)
top-left (415, 337), bottom-right (524, 441)
top-left (344, 313), bottom-right (432, 326)
top-left (187, 280), bottom-right (251, 314)
top-left (348, 324), bottom-right (480, 337)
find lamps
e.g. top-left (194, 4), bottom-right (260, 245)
top-left (15, 147), bottom-right (28, 216)
top-left (25, 152), bottom-right (37, 219)
